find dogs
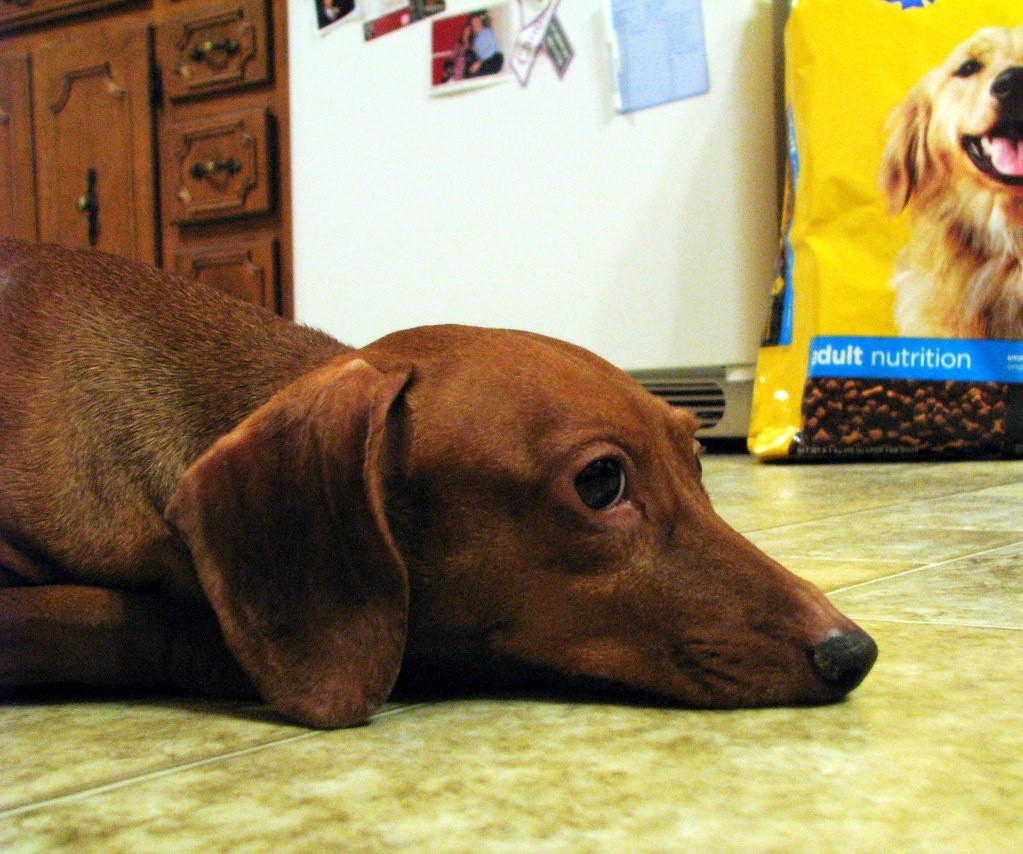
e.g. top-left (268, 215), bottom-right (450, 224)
top-left (0, 236), bottom-right (878, 730)
top-left (882, 23), bottom-right (1023, 339)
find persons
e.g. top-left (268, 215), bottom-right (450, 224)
top-left (433, 11), bottom-right (504, 83)
